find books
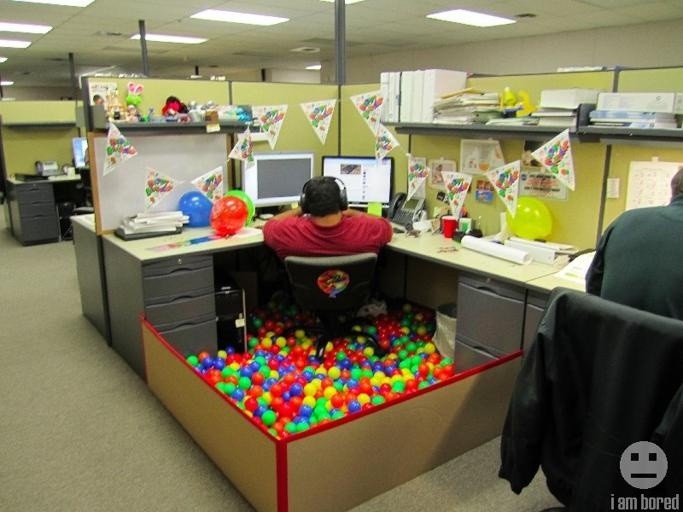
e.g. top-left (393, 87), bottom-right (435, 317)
top-left (112, 208), bottom-right (191, 241)
top-left (486, 118), bottom-right (538, 126)
top-left (380, 69), bottom-right (503, 126)
top-left (532, 90), bottom-right (682, 128)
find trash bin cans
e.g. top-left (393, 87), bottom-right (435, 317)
top-left (436, 302), bottom-right (457, 364)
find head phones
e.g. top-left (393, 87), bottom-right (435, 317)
top-left (297, 176), bottom-right (347, 207)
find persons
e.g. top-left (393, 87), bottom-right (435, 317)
top-left (583, 167), bottom-right (682, 322)
top-left (261, 175), bottom-right (392, 262)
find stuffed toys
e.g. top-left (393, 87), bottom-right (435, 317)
top-left (106, 80), bottom-right (218, 123)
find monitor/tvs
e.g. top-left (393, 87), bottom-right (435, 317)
top-left (72, 136), bottom-right (91, 174)
top-left (244, 151), bottom-right (315, 219)
top-left (322, 155), bottom-right (392, 207)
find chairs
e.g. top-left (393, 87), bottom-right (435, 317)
top-left (498, 287), bottom-right (683, 512)
top-left (72, 167), bottom-right (94, 215)
top-left (271, 253), bottom-right (386, 363)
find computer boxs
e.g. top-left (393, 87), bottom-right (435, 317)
top-left (213, 289), bottom-right (248, 355)
top-left (56, 202), bottom-right (77, 243)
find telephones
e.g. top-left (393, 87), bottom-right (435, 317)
top-left (387, 193), bottom-right (425, 229)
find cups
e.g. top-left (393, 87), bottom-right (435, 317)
top-left (444, 216), bottom-right (456, 237)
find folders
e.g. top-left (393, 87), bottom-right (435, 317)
top-left (15, 174), bottom-right (49, 181)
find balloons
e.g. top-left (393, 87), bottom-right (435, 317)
top-left (505, 195), bottom-right (553, 240)
top-left (178, 190), bottom-right (213, 229)
top-left (208, 195), bottom-right (249, 237)
top-left (186, 289), bottom-right (456, 440)
top-left (223, 189), bottom-right (255, 227)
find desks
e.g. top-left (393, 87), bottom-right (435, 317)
top-left (5, 174), bottom-right (81, 246)
top-left (70, 214), bottom-right (108, 343)
top-left (102, 226), bottom-right (560, 383)
top-left (522, 273), bottom-right (587, 352)
